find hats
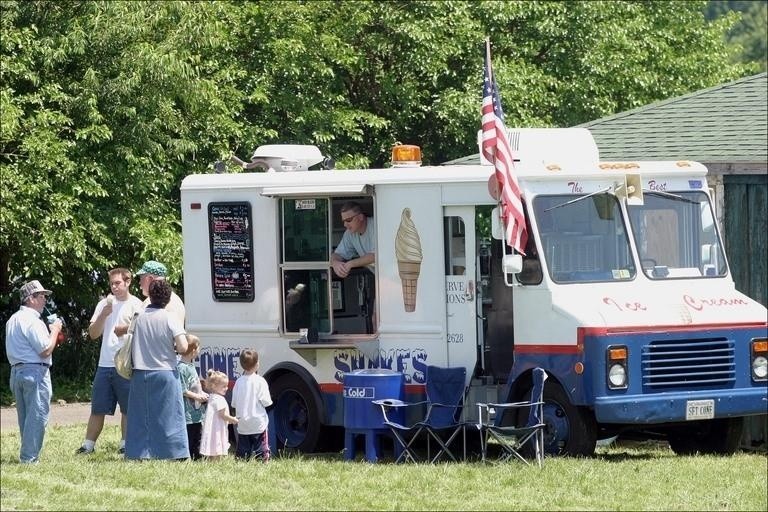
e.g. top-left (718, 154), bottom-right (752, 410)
top-left (136, 261), bottom-right (166, 276)
top-left (20, 280), bottom-right (52, 302)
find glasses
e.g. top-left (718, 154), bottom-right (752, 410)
top-left (341, 214), bottom-right (359, 223)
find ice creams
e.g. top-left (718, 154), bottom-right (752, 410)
top-left (295, 283), bottom-right (306, 293)
top-left (107, 293), bottom-right (113, 305)
top-left (395, 207), bottom-right (422, 312)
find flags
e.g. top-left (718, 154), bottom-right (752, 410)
top-left (481, 55), bottom-right (529, 258)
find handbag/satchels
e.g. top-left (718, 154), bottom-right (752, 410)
top-left (114, 333), bottom-right (133, 379)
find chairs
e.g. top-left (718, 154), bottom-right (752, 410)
top-left (472, 366), bottom-right (551, 462)
top-left (371, 366), bottom-right (469, 463)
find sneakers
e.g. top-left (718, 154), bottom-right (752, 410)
top-left (75, 446), bottom-right (94, 454)
top-left (118, 447), bottom-right (125, 455)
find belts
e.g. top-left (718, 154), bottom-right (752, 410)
top-left (12, 363), bottom-right (48, 367)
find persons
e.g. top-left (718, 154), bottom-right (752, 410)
top-left (74, 268), bottom-right (142, 455)
top-left (176, 334), bottom-right (210, 461)
top-left (113, 260), bottom-right (185, 365)
top-left (231, 349), bottom-right (273, 463)
top-left (330, 200), bottom-right (377, 334)
top-left (5, 279), bottom-right (63, 465)
top-left (199, 368), bottom-right (239, 461)
top-left (124, 280), bottom-right (191, 461)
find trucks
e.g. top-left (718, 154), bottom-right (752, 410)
top-left (176, 122), bottom-right (767, 460)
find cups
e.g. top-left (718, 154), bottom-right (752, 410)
top-left (46, 312), bottom-right (65, 344)
